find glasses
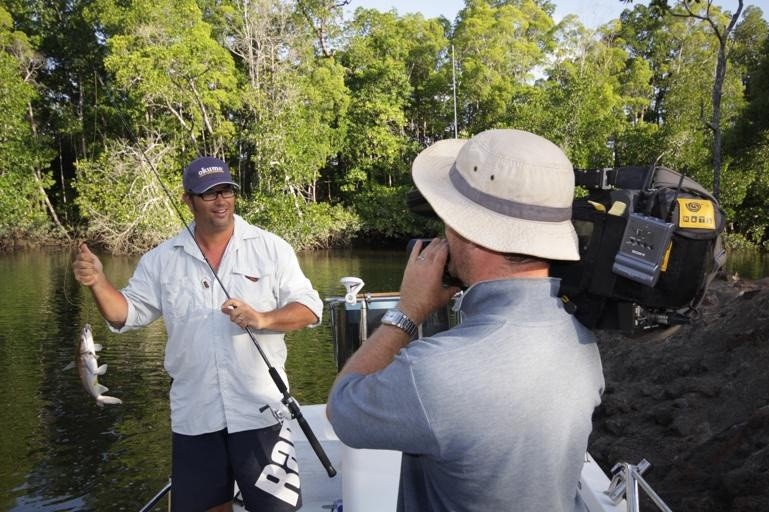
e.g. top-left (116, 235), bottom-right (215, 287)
top-left (182, 187), bottom-right (236, 204)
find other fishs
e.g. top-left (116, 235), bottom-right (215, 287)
top-left (62, 327), bottom-right (122, 408)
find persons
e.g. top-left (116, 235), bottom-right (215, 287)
top-left (325, 122), bottom-right (607, 512)
top-left (69, 155), bottom-right (326, 512)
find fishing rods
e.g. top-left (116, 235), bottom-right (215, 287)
top-left (95, 71), bottom-right (337, 478)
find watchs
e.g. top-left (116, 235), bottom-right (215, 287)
top-left (380, 309), bottom-right (417, 340)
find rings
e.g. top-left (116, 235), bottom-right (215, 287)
top-left (416, 256), bottom-right (426, 260)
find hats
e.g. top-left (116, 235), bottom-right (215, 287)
top-left (181, 156), bottom-right (243, 200)
top-left (409, 127), bottom-right (586, 264)
